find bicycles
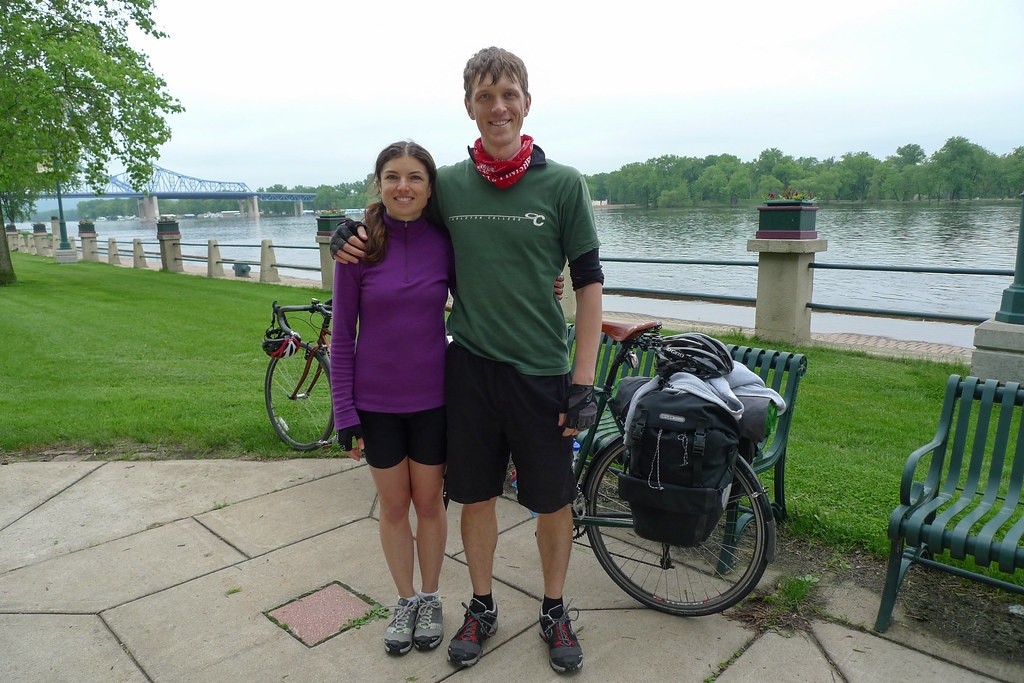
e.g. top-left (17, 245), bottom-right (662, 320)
top-left (264, 295), bottom-right (337, 451)
top-left (574, 319), bottom-right (776, 617)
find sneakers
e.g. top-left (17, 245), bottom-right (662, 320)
top-left (416, 593), bottom-right (443, 651)
top-left (539, 605), bottom-right (584, 672)
top-left (447, 598), bottom-right (499, 665)
top-left (384, 597), bottom-right (420, 655)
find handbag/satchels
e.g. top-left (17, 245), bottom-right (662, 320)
top-left (615, 377), bottom-right (772, 463)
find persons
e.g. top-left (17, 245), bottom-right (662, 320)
top-left (329, 47), bottom-right (606, 674)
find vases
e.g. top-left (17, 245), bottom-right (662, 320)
top-left (156, 221), bottom-right (181, 235)
top-left (755, 199), bottom-right (819, 239)
top-left (78, 223), bottom-right (96, 233)
top-left (316, 214), bottom-right (347, 236)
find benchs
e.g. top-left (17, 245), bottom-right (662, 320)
top-left (566, 324), bottom-right (808, 576)
top-left (873, 373), bottom-right (1024, 634)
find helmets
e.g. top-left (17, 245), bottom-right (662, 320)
top-left (263, 327), bottom-right (301, 359)
top-left (657, 331), bottom-right (734, 378)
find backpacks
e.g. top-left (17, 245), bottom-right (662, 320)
top-left (617, 393), bottom-right (739, 546)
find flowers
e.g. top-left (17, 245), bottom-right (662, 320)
top-left (78, 219), bottom-right (93, 225)
top-left (158, 216), bottom-right (176, 222)
top-left (761, 186), bottom-right (821, 202)
top-left (320, 208), bottom-right (348, 215)
